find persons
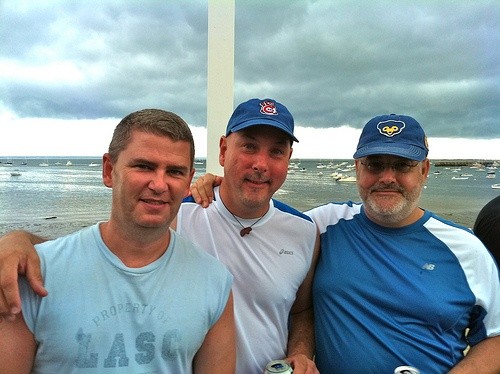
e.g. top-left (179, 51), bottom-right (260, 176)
top-left (473, 195), bottom-right (500, 270)
top-left (186, 114), bottom-right (500, 374)
top-left (0, 99), bottom-right (320, 374)
top-left (0, 109), bottom-right (236, 374)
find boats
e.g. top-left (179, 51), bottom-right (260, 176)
top-left (194, 162), bottom-right (204, 165)
top-left (428, 165), bottom-right (500, 190)
top-left (0, 160), bottom-right (101, 167)
top-left (10, 169), bottom-right (21, 176)
top-left (287, 159), bottom-right (358, 183)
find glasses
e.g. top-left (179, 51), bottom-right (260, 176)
top-left (358, 158), bottom-right (422, 173)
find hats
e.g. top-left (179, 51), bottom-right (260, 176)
top-left (354, 114), bottom-right (429, 161)
top-left (225, 99), bottom-right (299, 145)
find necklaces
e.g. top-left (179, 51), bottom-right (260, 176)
top-left (220, 196), bottom-right (270, 237)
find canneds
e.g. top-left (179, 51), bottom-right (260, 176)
top-left (264, 360), bottom-right (293, 374)
top-left (393, 365), bottom-right (420, 374)
